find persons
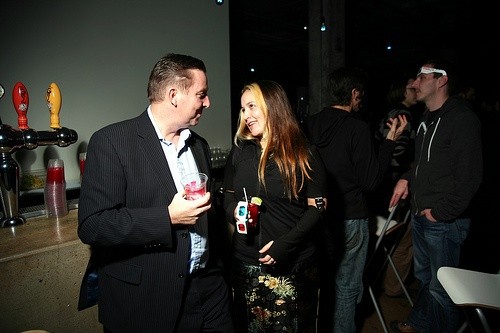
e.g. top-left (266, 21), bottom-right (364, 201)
top-left (214, 53), bottom-right (500, 333)
top-left (78, 52), bottom-right (240, 333)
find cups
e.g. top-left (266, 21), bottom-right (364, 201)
top-left (43, 159), bottom-right (68, 218)
top-left (80, 153), bottom-right (86, 176)
top-left (247, 203), bottom-right (258, 226)
top-left (180, 173), bottom-right (210, 215)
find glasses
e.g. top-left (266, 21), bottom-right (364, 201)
top-left (405, 87), bottom-right (415, 92)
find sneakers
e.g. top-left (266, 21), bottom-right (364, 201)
top-left (390, 320), bottom-right (415, 333)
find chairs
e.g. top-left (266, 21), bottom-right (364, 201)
top-left (437, 265), bottom-right (500, 333)
top-left (361, 198), bottom-right (413, 333)
